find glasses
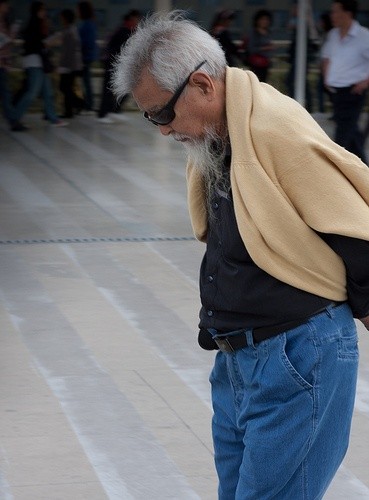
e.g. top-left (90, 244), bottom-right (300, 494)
top-left (144, 60), bottom-right (208, 125)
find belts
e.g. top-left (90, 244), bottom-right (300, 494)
top-left (206, 298), bottom-right (346, 352)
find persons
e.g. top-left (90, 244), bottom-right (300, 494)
top-left (0, 0), bottom-right (337, 132)
top-left (321, 0), bottom-right (369, 166)
top-left (111, 17), bottom-right (369, 500)
